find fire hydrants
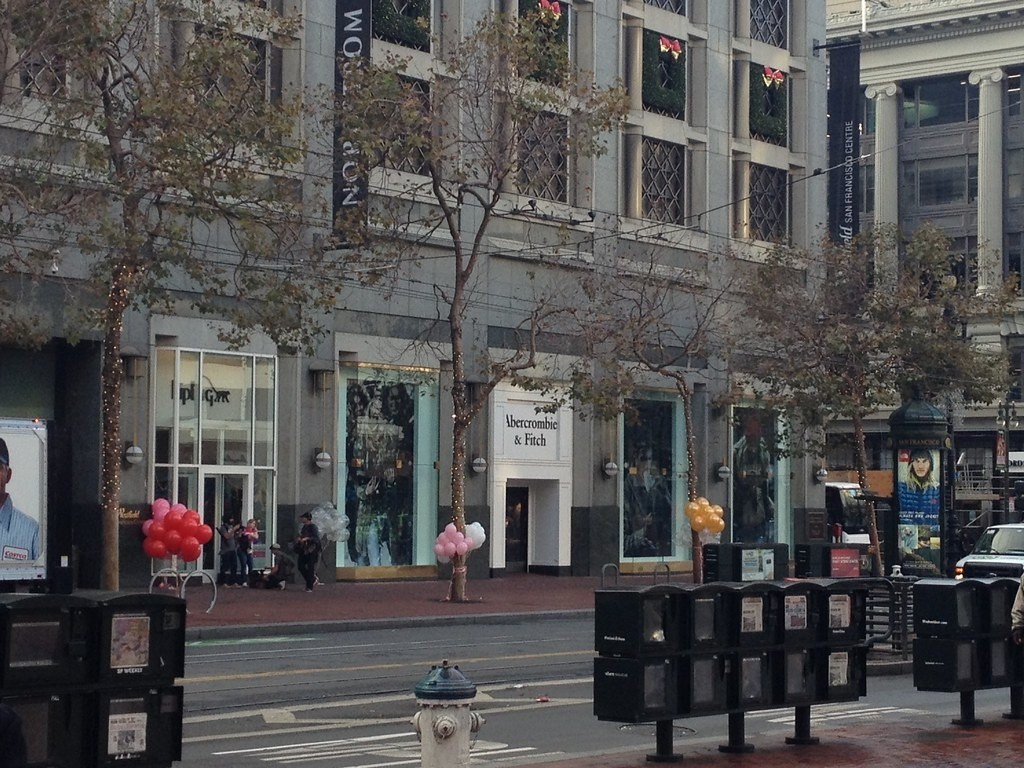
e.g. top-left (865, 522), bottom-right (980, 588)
top-left (409, 658), bottom-right (487, 768)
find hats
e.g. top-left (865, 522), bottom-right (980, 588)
top-left (270, 543), bottom-right (281, 549)
top-left (300, 512), bottom-right (312, 520)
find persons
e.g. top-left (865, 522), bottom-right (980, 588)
top-left (0, 438), bottom-right (40, 561)
top-left (1011, 572), bottom-right (1024, 645)
top-left (294, 512), bottom-right (320, 593)
top-left (269, 543), bottom-right (291, 590)
top-left (220, 514), bottom-right (258, 586)
top-left (898, 448), bottom-right (939, 525)
top-left (624, 423), bottom-right (672, 557)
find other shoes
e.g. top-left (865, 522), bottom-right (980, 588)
top-left (221, 583), bottom-right (230, 588)
top-left (242, 582), bottom-right (248, 587)
top-left (313, 576), bottom-right (318, 585)
top-left (305, 587), bottom-right (312, 592)
top-left (231, 583), bottom-right (240, 588)
top-left (279, 580), bottom-right (286, 590)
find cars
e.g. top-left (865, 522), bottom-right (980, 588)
top-left (953, 523), bottom-right (1024, 580)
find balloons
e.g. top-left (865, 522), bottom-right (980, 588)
top-left (298, 501), bottom-right (350, 546)
top-left (143, 499), bottom-right (211, 562)
top-left (685, 497), bottom-right (724, 533)
top-left (435, 521), bottom-right (486, 560)
